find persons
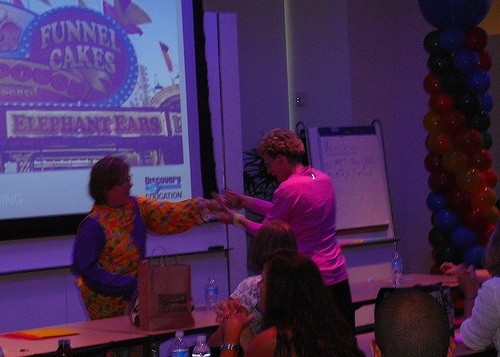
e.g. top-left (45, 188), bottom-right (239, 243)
top-left (207, 127), bottom-right (364, 357)
top-left (370, 225), bottom-right (500, 357)
top-left (73, 156), bottom-right (238, 357)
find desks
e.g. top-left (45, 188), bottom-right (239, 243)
top-left (1, 274), bottom-right (459, 357)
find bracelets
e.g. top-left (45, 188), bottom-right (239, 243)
top-left (233, 213), bottom-right (245, 228)
top-left (220, 344), bottom-right (240, 352)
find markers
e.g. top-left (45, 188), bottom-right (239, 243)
top-left (339, 237), bottom-right (386, 244)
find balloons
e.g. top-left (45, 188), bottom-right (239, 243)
top-left (419, 0), bottom-right (500, 271)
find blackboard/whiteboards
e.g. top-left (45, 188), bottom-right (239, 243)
top-left (306, 126), bottom-right (401, 248)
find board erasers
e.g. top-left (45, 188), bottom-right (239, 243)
top-left (209, 245), bottom-right (225, 251)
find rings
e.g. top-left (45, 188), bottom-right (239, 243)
top-left (225, 311), bottom-right (230, 317)
top-left (220, 318), bottom-right (225, 321)
top-left (234, 310), bottom-right (238, 314)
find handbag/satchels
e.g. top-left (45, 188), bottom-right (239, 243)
top-left (139, 247), bottom-right (195, 332)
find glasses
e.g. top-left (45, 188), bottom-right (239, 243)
top-left (116, 176), bottom-right (131, 186)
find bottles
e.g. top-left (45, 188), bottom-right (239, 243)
top-left (392, 250), bottom-right (403, 286)
top-left (192, 336), bottom-right (211, 357)
top-left (205, 273), bottom-right (218, 314)
top-left (168, 330), bottom-right (189, 357)
top-left (55, 340), bottom-right (72, 357)
